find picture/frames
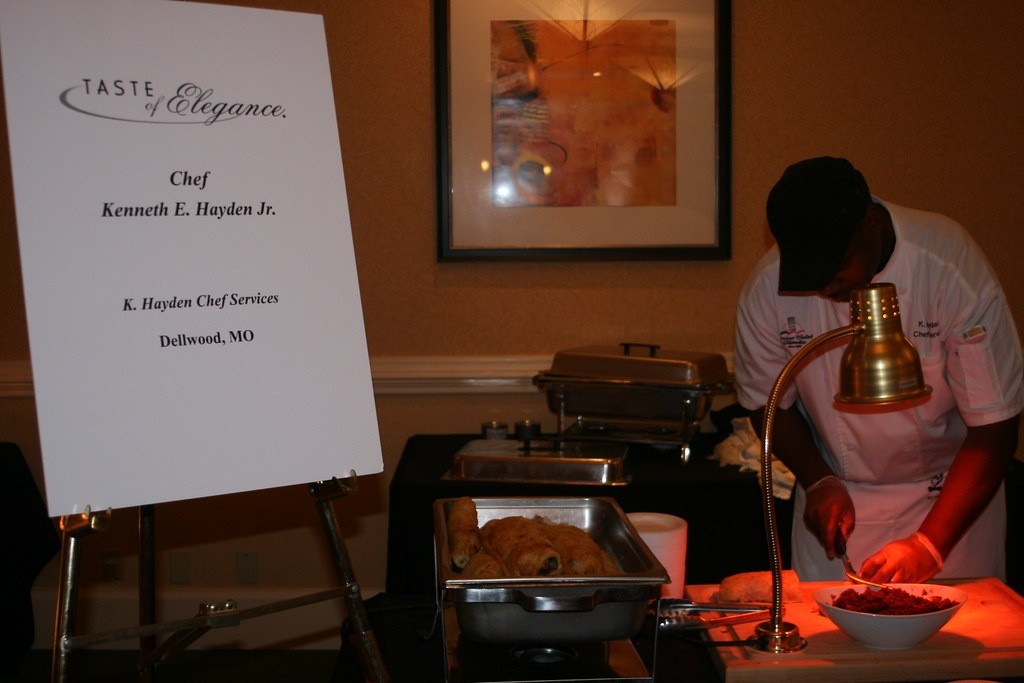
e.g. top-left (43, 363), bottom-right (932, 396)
top-left (431, 0), bottom-right (734, 265)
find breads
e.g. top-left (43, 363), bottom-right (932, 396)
top-left (447, 496), bottom-right (624, 580)
top-left (720, 569), bottom-right (805, 603)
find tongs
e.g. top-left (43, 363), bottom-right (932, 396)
top-left (648, 597), bottom-right (785, 633)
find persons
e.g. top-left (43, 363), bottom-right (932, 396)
top-left (733, 156), bottom-right (1023, 583)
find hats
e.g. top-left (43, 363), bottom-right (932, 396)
top-left (767, 156), bottom-right (873, 297)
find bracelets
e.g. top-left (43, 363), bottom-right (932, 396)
top-left (914, 531), bottom-right (944, 568)
top-left (806, 474), bottom-right (834, 492)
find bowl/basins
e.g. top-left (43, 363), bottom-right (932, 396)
top-left (815, 581), bottom-right (968, 650)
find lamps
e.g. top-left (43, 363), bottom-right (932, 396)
top-left (754, 282), bottom-right (932, 656)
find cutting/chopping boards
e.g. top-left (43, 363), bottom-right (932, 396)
top-left (683, 574), bottom-right (1024, 683)
